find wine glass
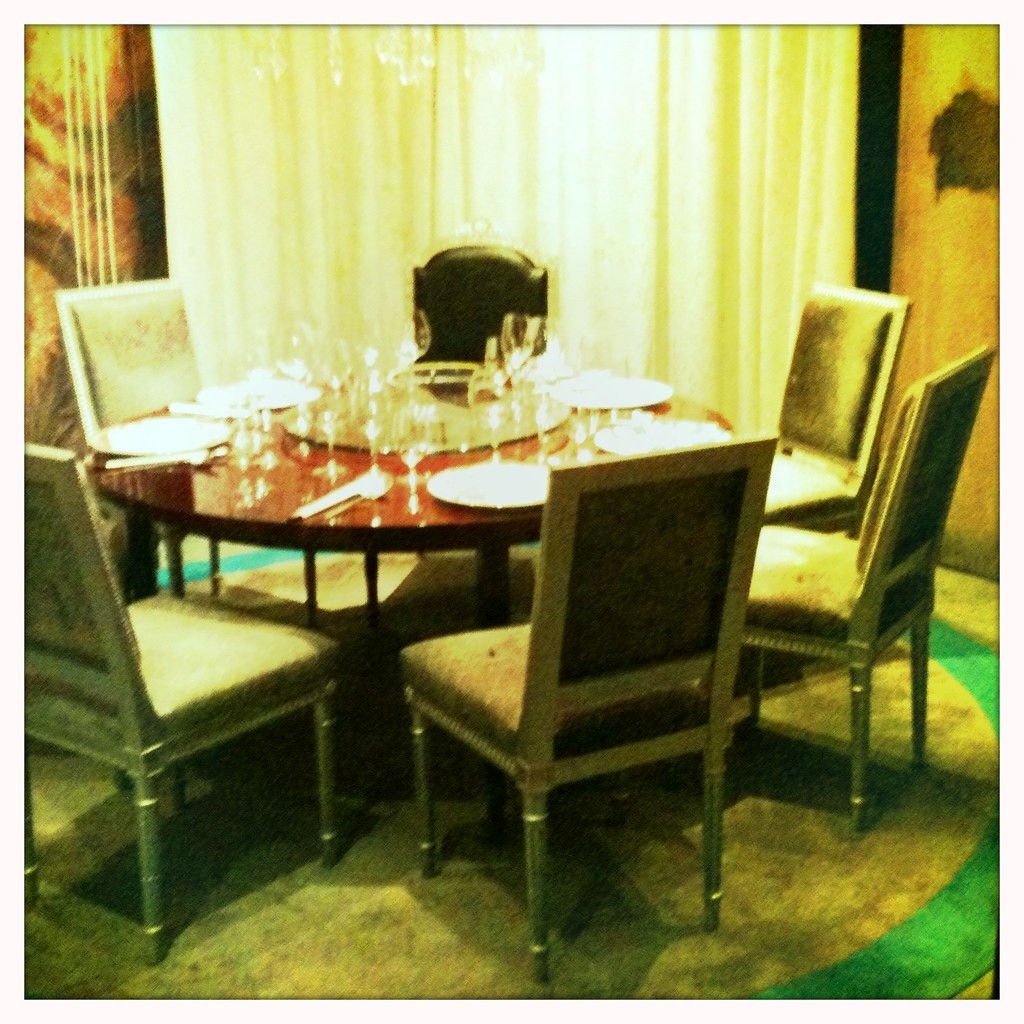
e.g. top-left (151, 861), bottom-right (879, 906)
top-left (221, 321), bottom-right (601, 486)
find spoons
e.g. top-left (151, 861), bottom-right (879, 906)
top-left (322, 471), bottom-right (394, 520)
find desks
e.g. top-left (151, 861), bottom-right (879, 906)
top-left (89, 377), bottom-right (736, 846)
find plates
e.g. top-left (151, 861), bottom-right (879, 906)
top-left (196, 380), bottom-right (322, 412)
top-left (89, 414), bottom-right (227, 455)
top-left (594, 418), bottom-right (730, 455)
top-left (549, 372), bottom-right (675, 410)
top-left (424, 460), bottom-right (555, 509)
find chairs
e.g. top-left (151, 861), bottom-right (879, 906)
top-left (410, 247), bottom-right (549, 373)
top-left (53, 279), bottom-right (379, 637)
top-left (667, 342), bottom-right (995, 830)
top-left (763, 283), bottom-right (915, 537)
top-left (25, 444), bottom-right (345, 967)
top-left (398, 432), bottom-right (781, 983)
